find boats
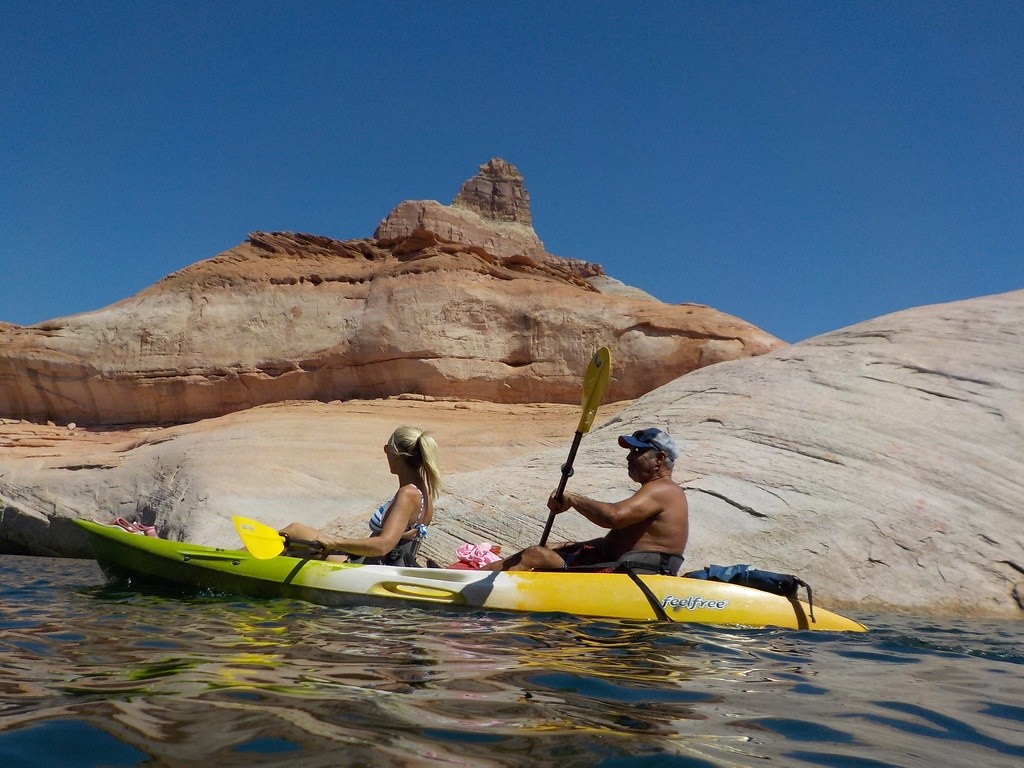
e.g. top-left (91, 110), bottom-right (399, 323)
top-left (44, 509), bottom-right (872, 636)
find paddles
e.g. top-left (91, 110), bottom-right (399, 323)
top-left (537, 343), bottom-right (614, 547)
top-left (230, 511), bottom-right (324, 563)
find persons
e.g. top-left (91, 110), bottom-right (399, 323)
top-left (238, 425), bottom-right (443, 568)
top-left (476, 428), bottom-right (689, 576)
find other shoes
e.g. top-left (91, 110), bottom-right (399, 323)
top-left (133, 521), bottom-right (157, 537)
top-left (113, 516), bottom-right (143, 534)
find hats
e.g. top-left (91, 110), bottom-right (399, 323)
top-left (618, 428), bottom-right (679, 461)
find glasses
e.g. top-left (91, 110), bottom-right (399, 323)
top-left (384, 445), bottom-right (391, 454)
